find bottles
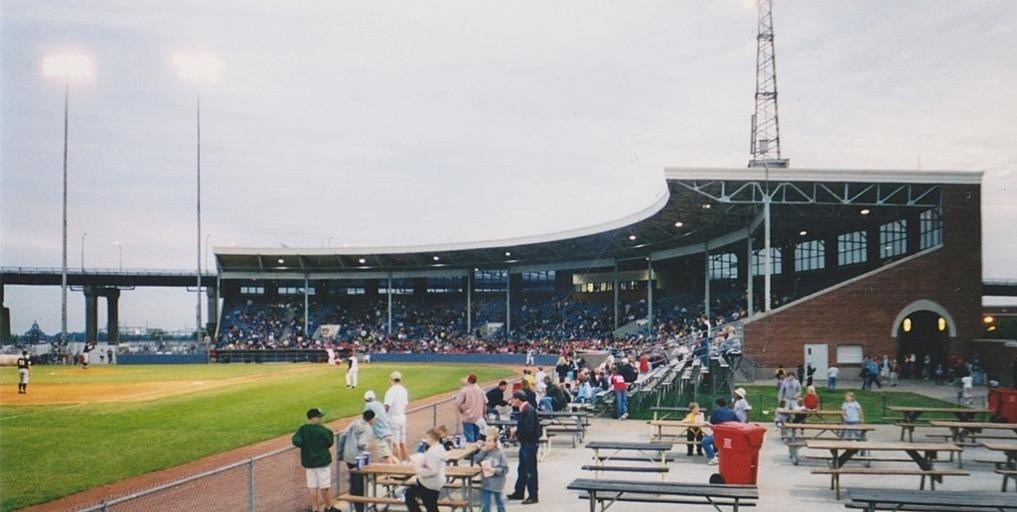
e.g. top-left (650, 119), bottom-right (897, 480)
top-left (487, 413), bottom-right (495, 423)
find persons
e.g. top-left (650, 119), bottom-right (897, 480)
top-left (520, 366), bottom-right (567, 412)
top-left (526, 346), bottom-right (536, 366)
top-left (860, 352), bottom-right (981, 388)
top-left (700, 398), bottom-right (742, 464)
top-left (439, 424), bottom-right (455, 452)
top-left (325, 346), bottom-right (336, 366)
top-left (100, 348), bottom-right (105, 362)
top-left (554, 315), bottom-right (740, 420)
top-left (17, 350), bottom-right (28, 393)
top-left (107, 348), bottom-right (113, 364)
top-left (826, 364), bottom-right (840, 391)
top-left (453, 374), bottom-right (510, 442)
top-left (363, 390), bottom-right (400, 465)
top-left (83, 341), bottom-right (92, 368)
top-left (774, 362), bottom-right (822, 424)
top-left (403, 427), bottom-right (445, 510)
top-left (383, 371), bottom-right (411, 461)
top-left (472, 435), bottom-right (510, 510)
top-left (961, 372), bottom-right (974, 405)
top-left (344, 352), bottom-right (357, 389)
top-left (733, 387), bottom-right (750, 424)
top-left (291, 407), bottom-right (339, 511)
top-left (219, 295), bottom-right (794, 355)
top-left (342, 411), bottom-right (375, 510)
top-left (685, 402), bottom-right (705, 456)
top-left (841, 391), bottom-right (865, 437)
top-left (506, 389), bottom-right (541, 504)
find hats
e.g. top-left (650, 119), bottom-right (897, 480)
top-left (390, 372), bottom-right (401, 380)
top-left (734, 388), bottom-right (746, 398)
top-left (364, 391), bottom-right (376, 400)
top-left (307, 409), bottom-right (326, 418)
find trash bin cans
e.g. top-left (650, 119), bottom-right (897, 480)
top-left (987, 387), bottom-right (1017, 423)
top-left (707, 420), bottom-right (767, 488)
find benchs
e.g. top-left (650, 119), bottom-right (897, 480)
top-left (336, 355), bottom-right (1017, 512)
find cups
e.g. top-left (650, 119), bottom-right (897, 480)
top-left (354, 456), bottom-right (365, 469)
top-left (361, 451), bottom-right (370, 464)
top-left (455, 433), bottom-right (462, 447)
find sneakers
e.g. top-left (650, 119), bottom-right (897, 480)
top-left (507, 491), bottom-right (524, 499)
top-left (522, 496), bottom-right (538, 504)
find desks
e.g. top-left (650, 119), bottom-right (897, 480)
top-left (584, 440), bottom-right (673, 473)
top-left (778, 407), bottom-right (847, 441)
top-left (981, 442), bottom-right (1017, 492)
top-left (886, 405), bottom-right (993, 443)
top-left (649, 406), bottom-right (708, 421)
top-left (359, 463), bottom-right (482, 511)
top-left (805, 438), bottom-right (963, 500)
top-left (784, 423), bottom-right (877, 466)
top-left (566, 478), bottom-right (760, 511)
top-left (929, 420), bottom-right (1017, 469)
top-left (845, 486), bottom-right (1017, 512)
top-left (647, 420), bottom-right (711, 456)
